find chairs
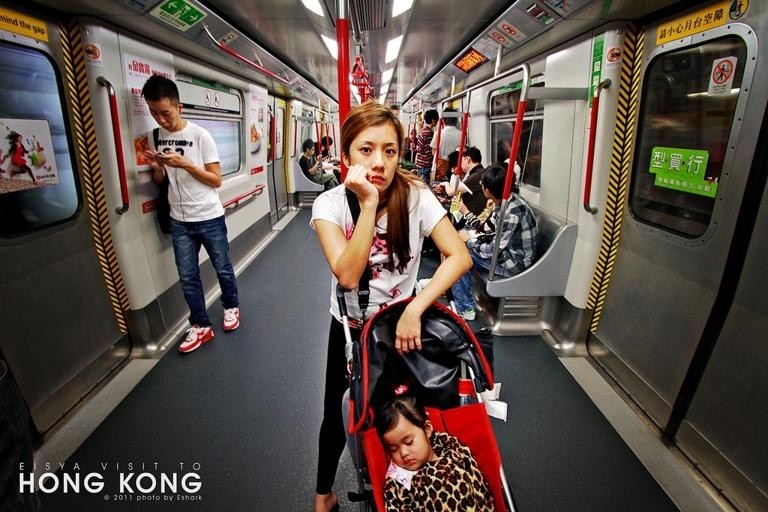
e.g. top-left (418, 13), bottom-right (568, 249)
top-left (295, 162), bottom-right (325, 208)
top-left (468, 198), bottom-right (578, 336)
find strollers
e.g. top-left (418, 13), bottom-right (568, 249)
top-left (336, 279), bottom-right (519, 512)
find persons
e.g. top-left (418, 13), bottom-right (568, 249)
top-left (415, 109), bottom-right (439, 184)
top-left (404, 129), bottom-right (418, 163)
top-left (140, 73), bottom-right (241, 353)
top-left (309, 99), bottom-right (474, 512)
top-left (431, 147), bottom-right (539, 322)
top-left (0, 131), bottom-right (39, 185)
top-left (430, 105), bottom-right (466, 181)
top-left (299, 136), bottom-right (341, 190)
top-left (375, 394), bottom-right (495, 512)
top-left (498, 122), bottom-right (542, 182)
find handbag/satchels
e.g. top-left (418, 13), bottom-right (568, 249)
top-left (156, 182), bottom-right (173, 233)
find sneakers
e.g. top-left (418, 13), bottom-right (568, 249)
top-left (179, 323), bottom-right (215, 354)
top-left (222, 307), bottom-right (241, 331)
top-left (445, 300), bottom-right (475, 321)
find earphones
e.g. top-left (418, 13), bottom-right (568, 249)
top-left (179, 110), bottom-right (181, 114)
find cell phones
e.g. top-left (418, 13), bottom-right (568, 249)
top-left (157, 152), bottom-right (166, 155)
